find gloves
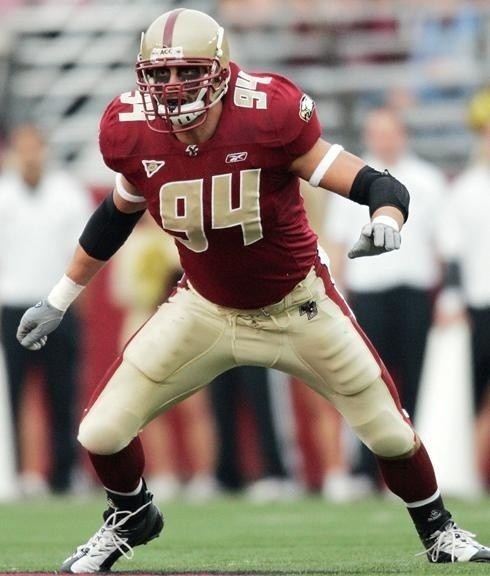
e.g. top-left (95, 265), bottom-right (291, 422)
top-left (16, 275), bottom-right (86, 350)
top-left (347, 216), bottom-right (402, 259)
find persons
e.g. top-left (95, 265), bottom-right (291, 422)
top-left (210, 367), bottom-right (307, 501)
top-left (321, 111), bottom-right (447, 499)
top-left (435, 89), bottom-right (489, 493)
top-left (105, 213), bottom-right (221, 502)
top-left (0, 119), bottom-right (97, 496)
top-left (15, 7), bottom-right (489, 575)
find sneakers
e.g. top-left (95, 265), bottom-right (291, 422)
top-left (424, 522), bottom-right (490, 562)
top-left (61, 494), bottom-right (164, 573)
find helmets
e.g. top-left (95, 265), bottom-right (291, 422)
top-left (136, 9), bottom-right (230, 133)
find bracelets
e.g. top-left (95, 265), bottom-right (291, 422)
top-left (372, 214), bottom-right (398, 232)
top-left (49, 273), bottom-right (86, 311)
